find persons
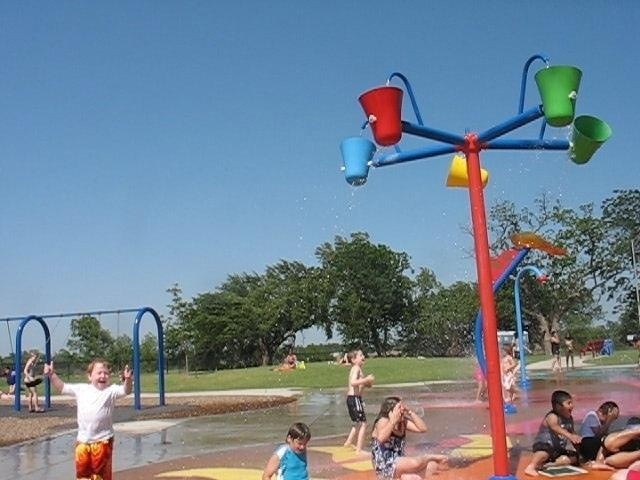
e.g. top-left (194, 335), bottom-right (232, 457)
top-left (512, 333), bottom-right (520, 358)
top-left (262, 423), bottom-right (311, 480)
top-left (371, 397), bottom-right (449, 480)
top-left (24, 350), bottom-right (45, 413)
top-left (474, 350), bottom-right (488, 402)
top-left (500, 343), bottom-right (520, 403)
top-left (524, 391), bottom-right (640, 477)
top-left (630, 336), bottom-right (640, 350)
top-left (286, 351), bottom-right (297, 368)
top-left (344, 348), bottom-right (375, 454)
top-left (0, 366), bottom-right (16, 401)
top-left (44, 357), bottom-right (132, 480)
top-left (550, 331), bottom-right (576, 373)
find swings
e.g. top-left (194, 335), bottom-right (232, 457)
top-left (94, 311), bottom-right (119, 385)
top-left (25, 314), bottom-right (79, 387)
top-left (0, 319), bottom-right (21, 384)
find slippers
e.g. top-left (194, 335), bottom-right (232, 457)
top-left (28, 409), bottom-right (45, 413)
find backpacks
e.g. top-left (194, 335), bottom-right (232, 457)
top-left (7, 375), bottom-right (15, 385)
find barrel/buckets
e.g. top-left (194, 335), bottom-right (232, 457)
top-left (534, 66), bottom-right (583, 127)
top-left (357, 84), bottom-right (404, 147)
top-left (445, 153), bottom-right (488, 190)
top-left (340, 136), bottom-right (377, 185)
top-left (569, 115), bottom-right (612, 164)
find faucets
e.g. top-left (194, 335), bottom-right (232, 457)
top-left (517, 53), bottom-right (548, 112)
top-left (387, 70), bottom-right (426, 127)
top-left (359, 120), bottom-right (403, 153)
